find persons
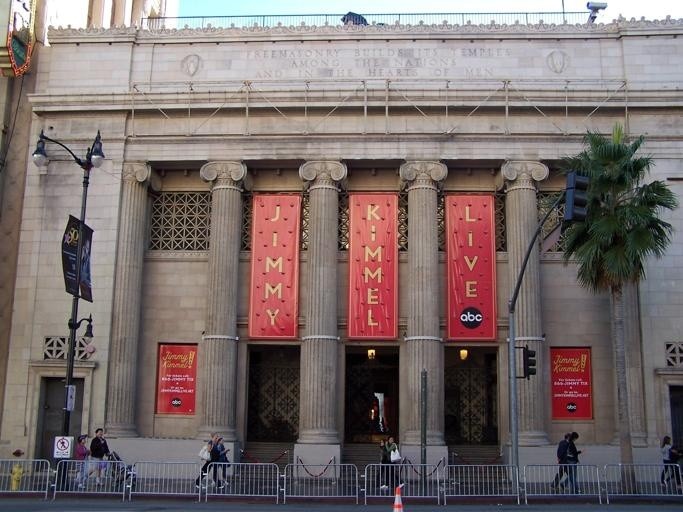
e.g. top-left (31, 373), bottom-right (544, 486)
top-left (377, 438), bottom-right (392, 489)
top-left (194, 430), bottom-right (222, 489)
top-left (72, 434), bottom-right (90, 489)
top-left (384, 435), bottom-right (404, 488)
top-left (559, 430), bottom-right (585, 494)
top-left (87, 427), bottom-right (110, 486)
top-left (664, 439), bottom-right (683, 488)
top-left (657, 435), bottom-right (675, 487)
top-left (212, 437), bottom-right (230, 489)
top-left (548, 432), bottom-right (571, 488)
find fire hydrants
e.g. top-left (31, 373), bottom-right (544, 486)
top-left (11, 463), bottom-right (25, 490)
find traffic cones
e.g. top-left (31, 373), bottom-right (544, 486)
top-left (392, 487), bottom-right (403, 512)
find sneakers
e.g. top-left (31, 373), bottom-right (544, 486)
top-left (196, 480), bottom-right (228, 488)
top-left (77, 482), bottom-right (104, 489)
top-left (400, 482), bottom-right (404, 488)
top-left (381, 485), bottom-right (388, 489)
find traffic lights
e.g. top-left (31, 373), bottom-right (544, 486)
top-left (523, 345), bottom-right (536, 381)
top-left (562, 172), bottom-right (590, 224)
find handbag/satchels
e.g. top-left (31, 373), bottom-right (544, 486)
top-left (198, 440), bottom-right (213, 462)
top-left (389, 443), bottom-right (401, 462)
top-left (225, 454), bottom-right (231, 468)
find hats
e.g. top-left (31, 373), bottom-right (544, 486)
top-left (78, 434), bottom-right (87, 441)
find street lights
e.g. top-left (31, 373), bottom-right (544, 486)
top-left (32, 129), bottom-right (105, 491)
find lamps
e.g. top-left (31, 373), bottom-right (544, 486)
top-left (459, 348), bottom-right (468, 360)
top-left (367, 347), bottom-right (376, 359)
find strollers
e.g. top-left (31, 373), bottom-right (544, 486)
top-left (108, 451), bottom-right (136, 495)
